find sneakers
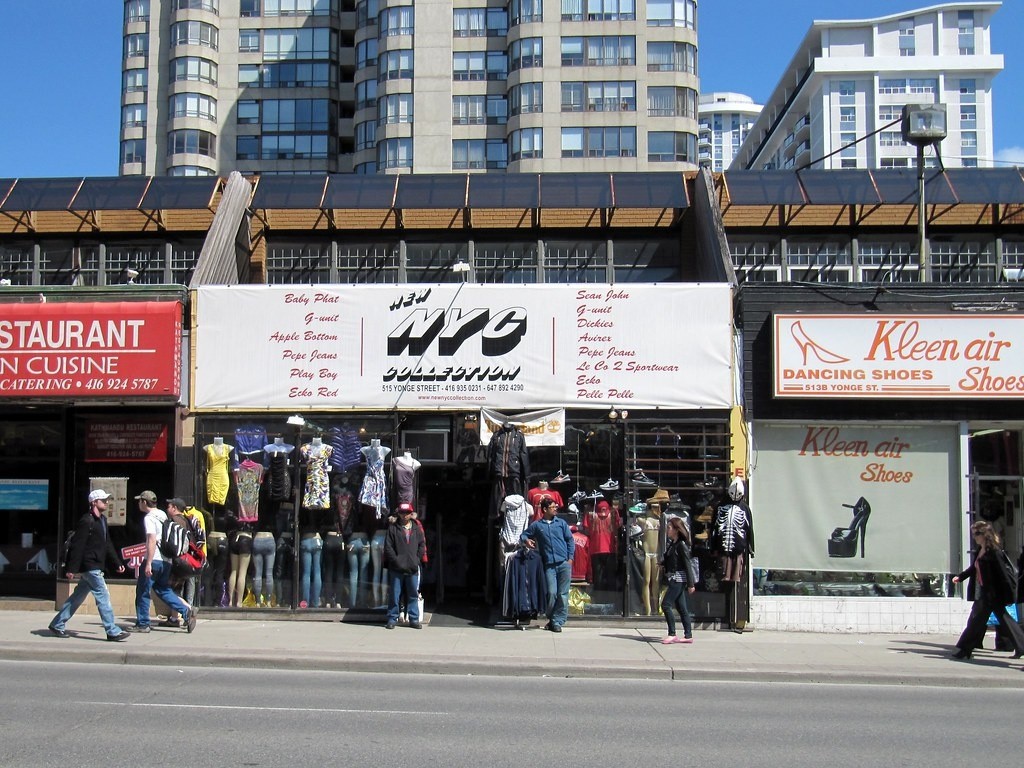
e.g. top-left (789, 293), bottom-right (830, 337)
top-left (549, 467), bottom-right (726, 539)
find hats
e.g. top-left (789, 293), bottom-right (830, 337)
top-left (88, 489), bottom-right (110, 503)
top-left (398, 502), bottom-right (414, 512)
top-left (135, 491), bottom-right (157, 503)
top-left (596, 501), bottom-right (610, 517)
top-left (166, 498), bottom-right (186, 512)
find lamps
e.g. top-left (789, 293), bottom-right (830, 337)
top-left (608, 405), bottom-right (628, 419)
top-left (898, 104), bottom-right (948, 282)
top-left (127, 270), bottom-right (138, 284)
top-left (0, 272), bottom-right (11, 286)
top-left (453, 261), bottom-right (471, 283)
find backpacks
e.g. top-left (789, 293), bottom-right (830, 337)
top-left (65, 516), bottom-right (95, 562)
top-left (986, 547), bottom-right (1020, 604)
top-left (145, 514), bottom-right (206, 579)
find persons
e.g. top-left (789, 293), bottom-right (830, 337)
top-left (655, 517), bottom-right (696, 644)
top-left (583, 502), bottom-right (620, 589)
top-left (175, 504), bottom-right (205, 606)
top-left (203, 508), bottom-right (428, 626)
top-left (487, 422), bottom-right (529, 514)
top-left (631, 489), bottom-right (699, 616)
top-left (712, 475), bottom-right (754, 584)
top-left (520, 498), bottom-right (575, 632)
top-left (124, 490), bottom-right (197, 633)
top-left (262, 436), bottom-right (296, 499)
top-left (391, 451), bottom-right (422, 505)
top-left (501, 493), bottom-right (534, 566)
top-left (385, 503), bottom-right (424, 629)
top-left (47, 489), bottom-right (131, 641)
top-left (299, 435), bottom-right (333, 510)
top-left (360, 437), bottom-right (392, 518)
top-left (203, 436), bottom-right (235, 506)
top-left (526, 479), bottom-right (564, 521)
top-left (158, 497), bottom-right (196, 628)
top-left (951, 521), bottom-right (1024, 660)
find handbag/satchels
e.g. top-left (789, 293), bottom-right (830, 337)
top-left (673, 541), bottom-right (700, 584)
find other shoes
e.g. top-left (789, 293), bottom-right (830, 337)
top-left (662, 636), bottom-right (678, 644)
top-left (674, 638), bottom-right (693, 643)
top-left (546, 621), bottom-right (561, 631)
top-left (386, 622), bottom-right (395, 629)
top-left (107, 632), bottom-right (130, 641)
top-left (409, 622), bottom-right (422, 629)
top-left (48, 626), bottom-right (69, 638)
top-left (126, 605), bottom-right (199, 633)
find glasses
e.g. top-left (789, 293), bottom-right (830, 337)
top-left (972, 531), bottom-right (985, 536)
top-left (399, 510), bottom-right (413, 515)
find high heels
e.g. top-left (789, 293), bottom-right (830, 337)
top-left (828, 496), bottom-right (871, 558)
top-left (1009, 650), bottom-right (1024, 659)
top-left (952, 648), bottom-right (972, 659)
